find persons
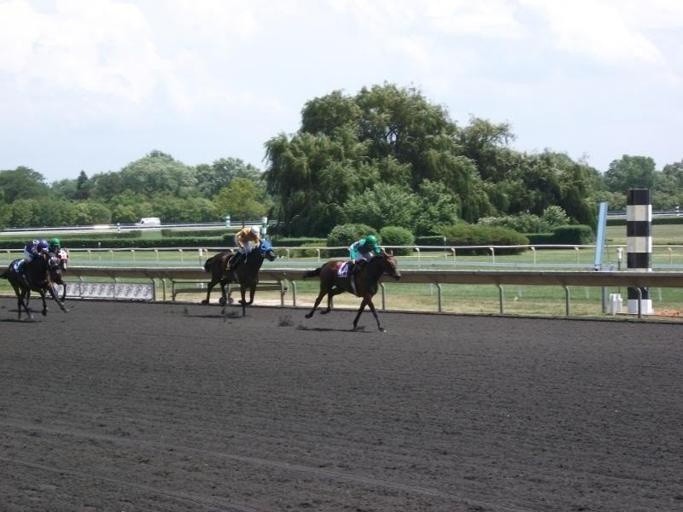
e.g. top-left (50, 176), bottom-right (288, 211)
top-left (234, 225), bottom-right (259, 252)
top-left (47, 238), bottom-right (60, 252)
top-left (21, 240), bottom-right (47, 263)
top-left (346, 235), bottom-right (386, 265)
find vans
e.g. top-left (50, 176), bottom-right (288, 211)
top-left (132, 217), bottom-right (161, 228)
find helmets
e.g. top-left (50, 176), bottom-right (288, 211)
top-left (252, 227), bottom-right (258, 236)
top-left (38, 238), bottom-right (47, 248)
top-left (366, 235), bottom-right (376, 245)
top-left (49, 238), bottom-right (60, 246)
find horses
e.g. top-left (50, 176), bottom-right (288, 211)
top-left (26, 246), bottom-right (69, 306)
top-left (201, 239), bottom-right (277, 316)
top-left (302, 249), bottom-right (402, 332)
top-left (0, 250), bottom-right (71, 321)
